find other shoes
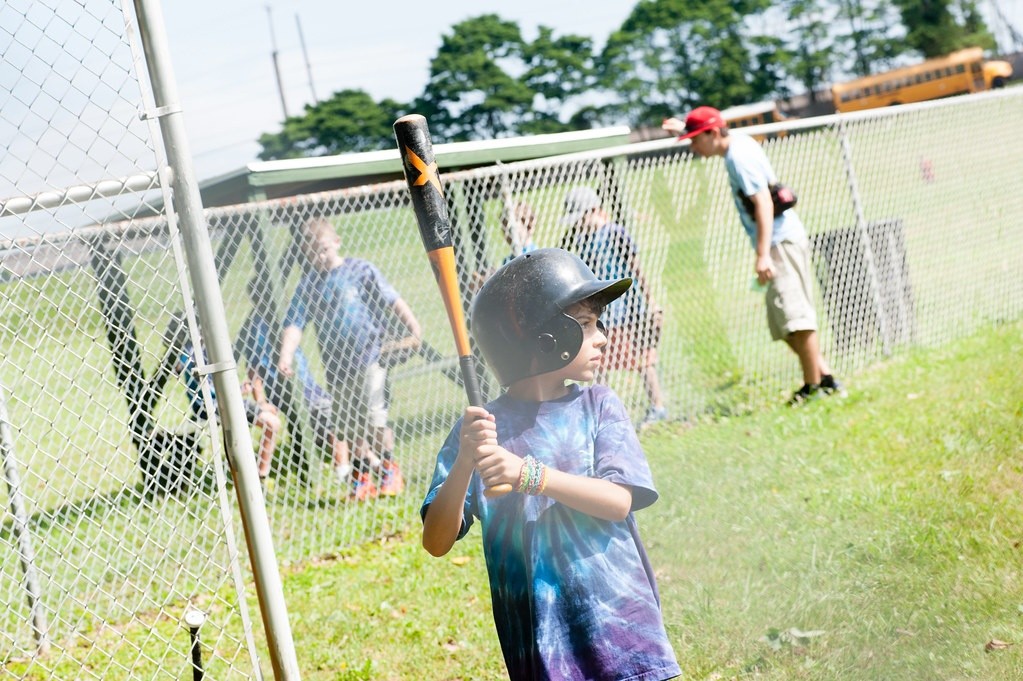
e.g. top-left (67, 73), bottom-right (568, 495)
top-left (341, 472), bottom-right (379, 501)
top-left (636, 405), bottom-right (669, 430)
top-left (816, 374), bottom-right (842, 395)
top-left (379, 459), bottom-right (405, 498)
top-left (784, 383), bottom-right (823, 410)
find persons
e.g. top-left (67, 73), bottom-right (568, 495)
top-left (159, 311), bottom-right (281, 483)
top-left (661, 106), bottom-right (843, 409)
top-left (235, 277), bottom-right (382, 483)
top-left (559, 183), bottom-right (671, 427)
top-left (497, 201), bottom-right (541, 266)
top-left (278, 219), bottom-right (426, 500)
top-left (420, 247), bottom-right (685, 681)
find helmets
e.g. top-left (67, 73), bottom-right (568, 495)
top-left (469, 247), bottom-right (632, 387)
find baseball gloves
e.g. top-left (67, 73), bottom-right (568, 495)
top-left (735, 183), bottom-right (798, 223)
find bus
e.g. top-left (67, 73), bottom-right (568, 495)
top-left (715, 100), bottom-right (795, 142)
top-left (829, 47), bottom-right (1014, 114)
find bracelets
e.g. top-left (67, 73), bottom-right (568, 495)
top-left (513, 454), bottom-right (547, 496)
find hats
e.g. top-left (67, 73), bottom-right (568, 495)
top-left (675, 106), bottom-right (727, 141)
top-left (559, 186), bottom-right (602, 224)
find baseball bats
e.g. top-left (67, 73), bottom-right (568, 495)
top-left (184, 609), bottom-right (207, 681)
top-left (388, 109), bottom-right (514, 500)
